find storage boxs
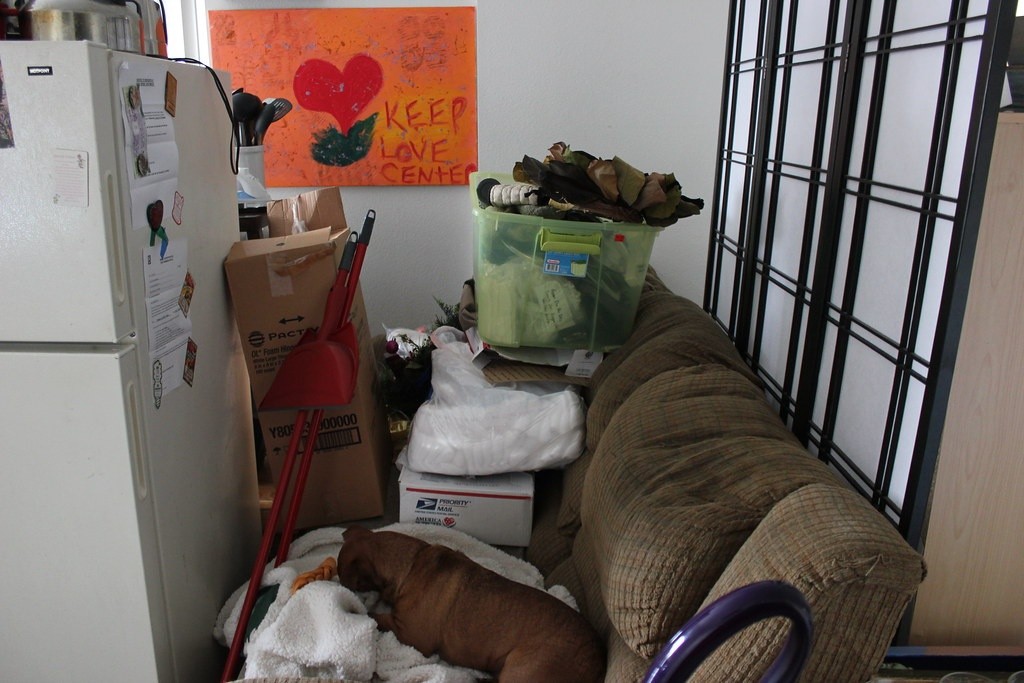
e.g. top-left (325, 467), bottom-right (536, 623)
top-left (222, 186), bottom-right (391, 530)
top-left (397, 466), bottom-right (534, 547)
top-left (471, 171), bottom-right (665, 354)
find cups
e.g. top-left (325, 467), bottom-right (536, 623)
top-left (230, 145), bottom-right (265, 205)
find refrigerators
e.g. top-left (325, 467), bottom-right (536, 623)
top-left (0, 40), bottom-right (263, 683)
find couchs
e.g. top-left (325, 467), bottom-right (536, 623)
top-left (238, 265), bottom-right (928, 682)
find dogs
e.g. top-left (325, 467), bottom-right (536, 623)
top-left (337, 524), bottom-right (606, 683)
